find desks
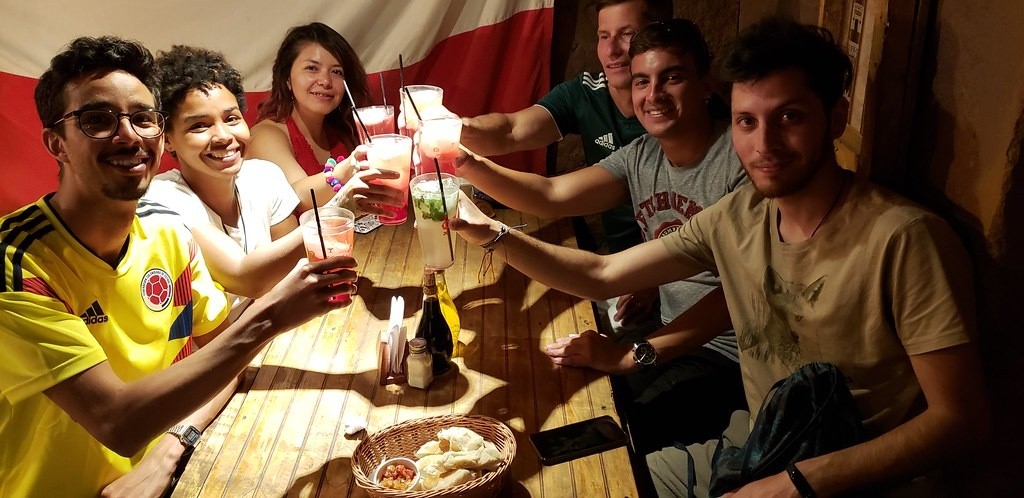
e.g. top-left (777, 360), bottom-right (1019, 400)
top-left (169, 184), bottom-right (658, 498)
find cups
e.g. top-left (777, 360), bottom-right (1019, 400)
top-left (373, 457), bottom-right (421, 491)
top-left (400, 85), bottom-right (444, 169)
top-left (365, 134), bottom-right (412, 226)
top-left (352, 105), bottom-right (394, 160)
top-left (417, 115), bottom-right (462, 176)
top-left (299, 207), bottom-right (355, 302)
top-left (409, 171), bottom-right (460, 269)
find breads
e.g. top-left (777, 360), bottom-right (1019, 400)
top-left (414, 426), bottom-right (503, 490)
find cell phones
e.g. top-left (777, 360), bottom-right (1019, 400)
top-left (529, 415), bottom-right (629, 466)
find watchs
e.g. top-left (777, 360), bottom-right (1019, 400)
top-left (632, 338), bottom-right (658, 369)
top-left (165, 425), bottom-right (203, 456)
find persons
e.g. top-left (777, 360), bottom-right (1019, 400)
top-left (413, 15), bottom-right (748, 455)
top-left (135, 42), bottom-right (406, 351)
top-left (0, 35), bottom-right (358, 498)
top-left (398, 0), bottom-right (674, 251)
top-left (409, 13), bottom-right (988, 497)
top-left (242, 21), bottom-right (377, 218)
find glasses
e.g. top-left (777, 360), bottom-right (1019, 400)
top-left (48, 107), bottom-right (173, 140)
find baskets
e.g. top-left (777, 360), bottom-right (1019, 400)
top-left (351, 414), bottom-right (518, 498)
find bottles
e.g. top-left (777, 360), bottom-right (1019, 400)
top-left (405, 338), bottom-right (433, 391)
top-left (435, 269), bottom-right (460, 359)
top-left (415, 269), bottom-right (453, 377)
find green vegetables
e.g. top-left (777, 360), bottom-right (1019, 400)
top-left (412, 187), bottom-right (447, 222)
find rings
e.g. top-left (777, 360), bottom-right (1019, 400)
top-left (635, 302), bottom-right (641, 307)
top-left (631, 295), bottom-right (637, 301)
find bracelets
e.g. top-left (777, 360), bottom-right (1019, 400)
top-left (323, 155), bottom-right (345, 192)
top-left (477, 220), bottom-right (527, 284)
top-left (785, 463), bottom-right (817, 498)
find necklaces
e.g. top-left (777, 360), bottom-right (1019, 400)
top-left (809, 169), bottom-right (846, 238)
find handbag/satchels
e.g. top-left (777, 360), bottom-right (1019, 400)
top-left (708, 361), bottom-right (882, 498)
